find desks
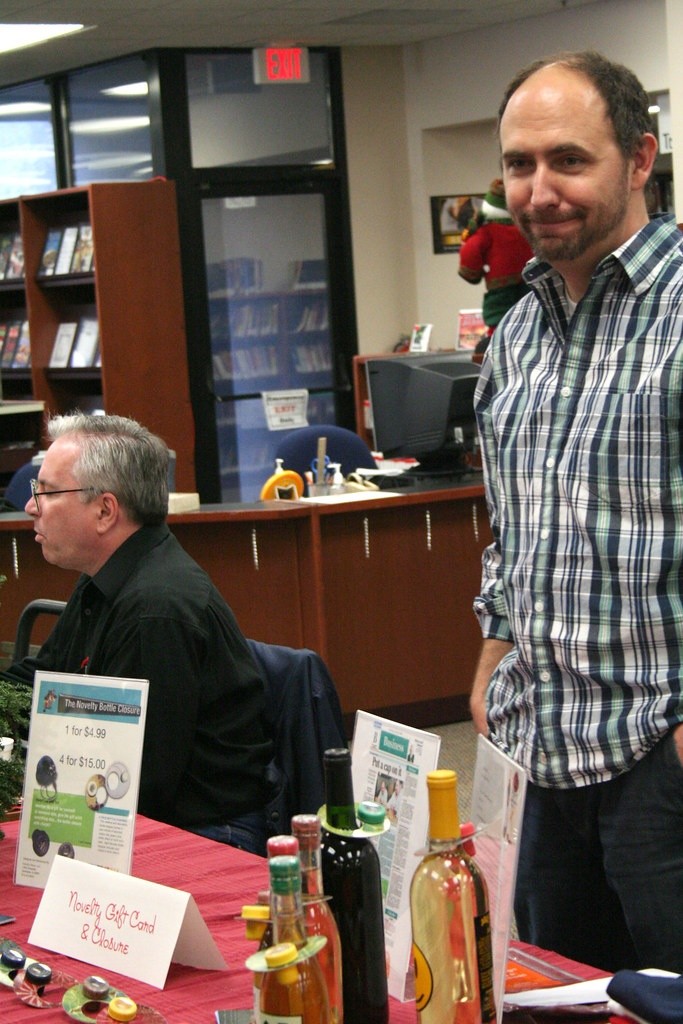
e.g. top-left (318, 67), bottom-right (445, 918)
top-left (0, 474), bottom-right (492, 740)
top-left (0, 789), bottom-right (639, 1024)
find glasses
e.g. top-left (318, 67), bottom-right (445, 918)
top-left (30, 478), bottom-right (104, 512)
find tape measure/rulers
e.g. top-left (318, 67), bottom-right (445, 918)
top-left (316, 437), bottom-right (327, 485)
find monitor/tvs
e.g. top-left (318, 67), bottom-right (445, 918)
top-left (366, 352), bottom-right (482, 477)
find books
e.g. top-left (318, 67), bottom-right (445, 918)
top-left (205, 257), bottom-right (328, 298)
top-left (208, 303), bottom-right (330, 338)
top-left (0, 224), bottom-right (97, 280)
top-left (214, 395), bottom-right (336, 470)
top-left (213, 340), bottom-right (330, 382)
top-left (1, 319), bottom-right (103, 369)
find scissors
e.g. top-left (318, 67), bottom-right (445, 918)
top-left (311, 455), bottom-right (330, 483)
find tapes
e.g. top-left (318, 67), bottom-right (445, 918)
top-left (346, 472), bottom-right (364, 485)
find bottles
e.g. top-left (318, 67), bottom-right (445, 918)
top-left (292, 814), bottom-right (343, 1024)
top-left (321, 749), bottom-right (389, 1024)
top-left (462, 849), bottom-right (498, 1024)
top-left (254, 836), bottom-right (330, 1024)
top-left (410, 770), bottom-right (480, 1024)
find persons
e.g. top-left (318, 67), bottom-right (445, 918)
top-left (0, 412), bottom-right (286, 857)
top-left (456, 178), bottom-right (535, 364)
top-left (467, 50), bottom-right (683, 977)
top-left (375, 779), bottom-right (403, 825)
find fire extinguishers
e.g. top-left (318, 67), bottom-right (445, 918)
top-left (392, 336), bottom-right (410, 353)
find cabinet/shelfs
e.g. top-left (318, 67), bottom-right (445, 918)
top-left (0, 177), bottom-right (195, 509)
